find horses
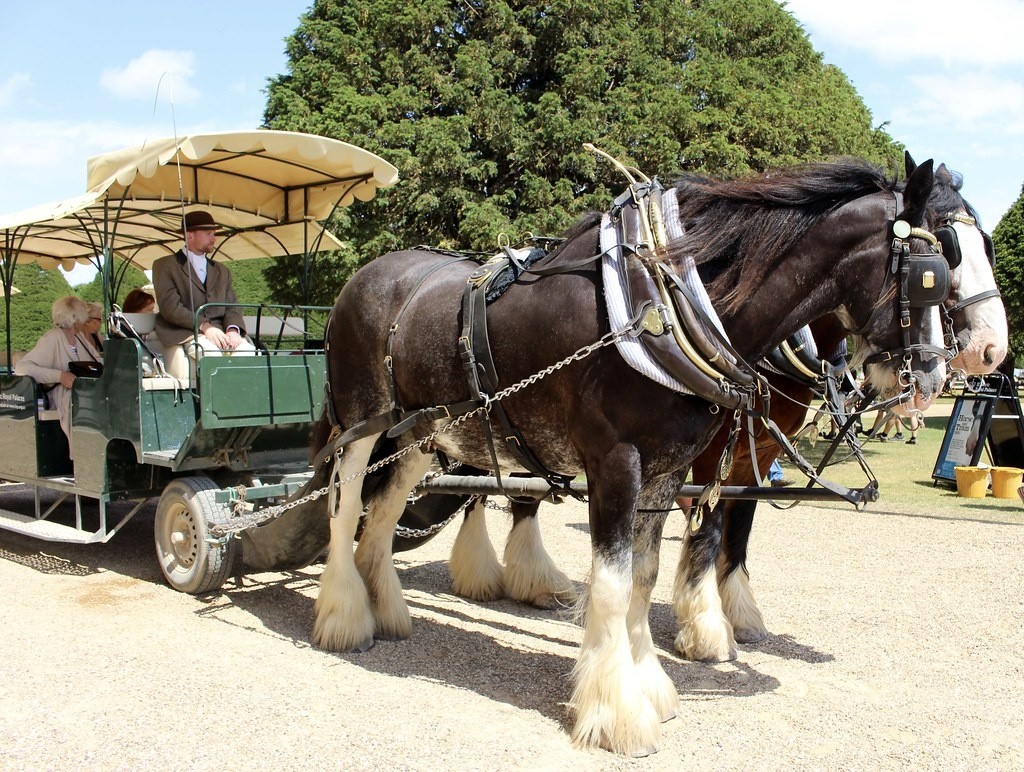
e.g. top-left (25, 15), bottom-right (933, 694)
top-left (446, 149), bottom-right (1008, 665)
top-left (311, 157), bottom-right (951, 758)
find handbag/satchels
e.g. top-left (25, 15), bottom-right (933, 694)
top-left (68, 361), bottom-right (103, 378)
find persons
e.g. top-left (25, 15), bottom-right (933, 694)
top-left (766, 458), bottom-right (796, 487)
top-left (79, 301), bottom-right (104, 353)
top-left (152, 210), bottom-right (255, 360)
top-left (824, 418), bottom-right (837, 439)
top-left (122, 289), bottom-right (155, 344)
top-left (862, 409), bottom-right (918, 445)
top-left (14, 294), bottom-right (104, 462)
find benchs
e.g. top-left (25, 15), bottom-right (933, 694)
top-left (0, 366), bottom-right (66, 478)
top-left (70, 335), bottom-right (138, 490)
top-left (109, 312), bottom-right (308, 451)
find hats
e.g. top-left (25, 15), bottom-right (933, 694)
top-left (175, 211), bottom-right (223, 233)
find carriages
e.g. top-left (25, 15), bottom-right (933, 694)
top-left (0, 127), bottom-right (1009, 758)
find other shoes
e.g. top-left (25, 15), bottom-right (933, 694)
top-left (888, 434), bottom-right (906, 442)
top-left (862, 429), bottom-right (875, 438)
top-left (905, 439), bottom-right (918, 444)
top-left (876, 432), bottom-right (887, 442)
top-left (823, 432), bottom-right (837, 440)
top-left (770, 478), bottom-right (797, 487)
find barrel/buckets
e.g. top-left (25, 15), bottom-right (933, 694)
top-left (954, 466), bottom-right (991, 498)
top-left (991, 466), bottom-right (1024, 498)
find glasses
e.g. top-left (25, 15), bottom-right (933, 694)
top-left (90, 316), bottom-right (103, 321)
